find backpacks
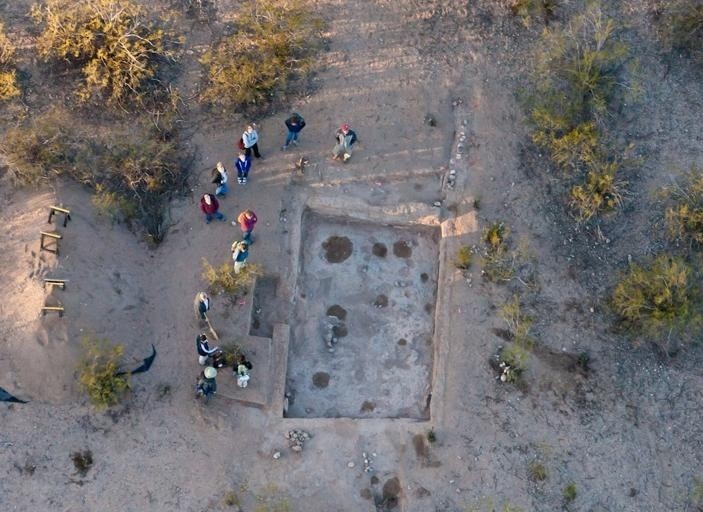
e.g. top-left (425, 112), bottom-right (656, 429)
top-left (194, 379), bottom-right (209, 399)
top-left (231, 240), bottom-right (241, 253)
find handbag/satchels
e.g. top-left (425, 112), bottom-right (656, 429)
top-left (239, 138), bottom-right (245, 150)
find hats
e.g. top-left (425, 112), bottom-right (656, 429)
top-left (204, 366), bottom-right (217, 379)
top-left (341, 123), bottom-right (350, 130)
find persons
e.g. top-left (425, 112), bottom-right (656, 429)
top-left (330, 123), bottom-right (357, 162)
top-left (199, 192), bottom-right (226, 226)
top-left (195, 332), bottom-right (218, 366)
top-left (208, 160), bottom-right (229, 200)
top-left (241, 122), bottom-right (262, 161)
top-left (238, 209), bottom-right (257, 246)
top-left (196, 290), bottom-right (210, 321)
top-left (230, 354), bottom-right (255, 389)
top-left (231, 239), bottom-right (249, 274)
top-left (196, 366), bottom-right (216, 403)
top-left (280, 112), bottom-right (306, 152)
top-left (232, 151), bottom-right (251, 186)
